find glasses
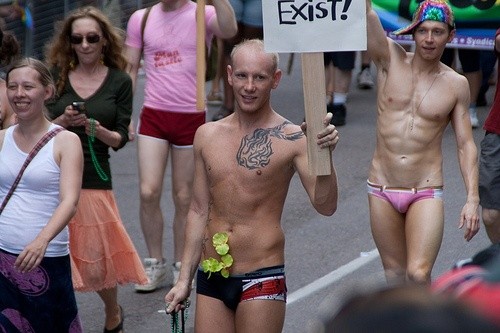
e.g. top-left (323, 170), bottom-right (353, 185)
top-left (70, 34), bottom-right (105, 44)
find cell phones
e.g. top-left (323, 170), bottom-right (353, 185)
top-left (73, 102), bottom-right (87, 115)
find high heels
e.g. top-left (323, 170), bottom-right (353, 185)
top-left (103, 305), bottom-right (124, 333)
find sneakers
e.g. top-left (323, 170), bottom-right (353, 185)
top-left (134, 258), bottom-right (173, 291)
top-left (170, 261), bottom-right (195, 289)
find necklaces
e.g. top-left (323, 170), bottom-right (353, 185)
top-left (88, 118), bottom-right (109, 181)
top-left (166, 300), bottom-right (188, 333)
top-left (411, 61), bottom-right (437, 129)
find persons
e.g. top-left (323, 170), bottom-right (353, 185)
top-left (441, 47), bottom-right (498, 129)
top-left (325, 51), bottom-right (373, 126)
top-left (324, 286), bottom-right (500, 333)
top-left (479, 28), bottom-right (500, 245)
top-left (165, 39), bottom-right (339, 333)
top-left (46, 7), bottom-right (148, 333)
top-left (206, 0), bottom-right (264, 122)
top-left (361, 0), bottom-right (481, 287)
top-left (0, 29), bottom-right (18, 128)
top-left (0, 58), bottom-right (83, 333)
top-left (125, 0), bottom-right (238, 290)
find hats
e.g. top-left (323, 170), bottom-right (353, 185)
top-left (390, 0), bottom-right (456, 36)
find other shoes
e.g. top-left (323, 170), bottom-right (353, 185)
top-left (357, 68), bottom-right (373, 89)
top-left (205, 92), bottom-right (223, 104)
top-left (469, 107), bottom-right (478, 126)
top-left (327, 103), bottom-right (347, 127)
